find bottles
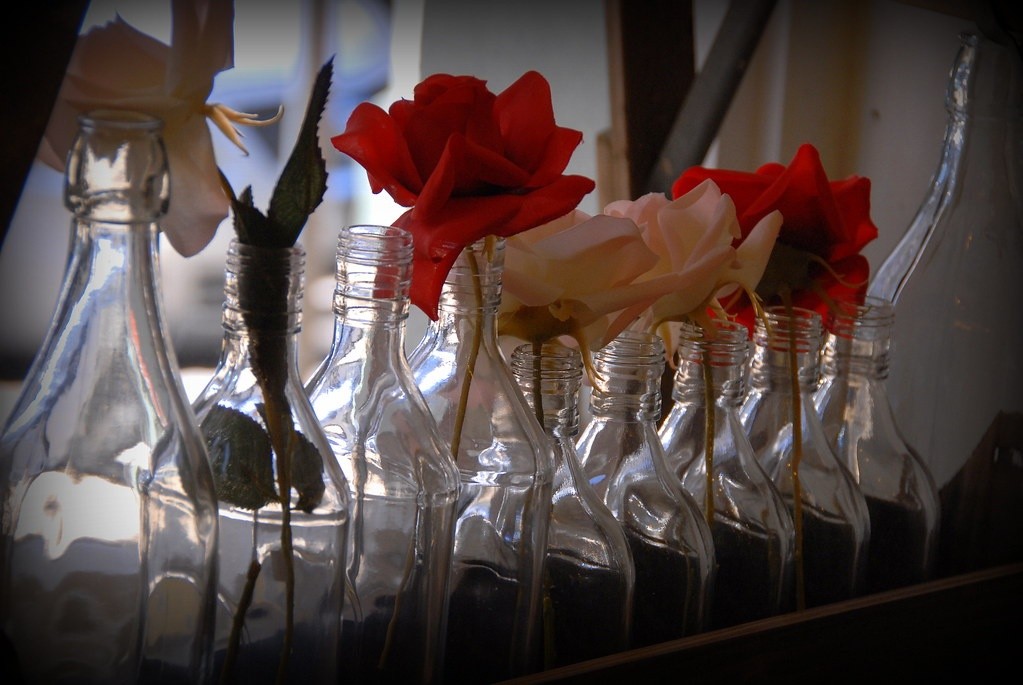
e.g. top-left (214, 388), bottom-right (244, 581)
top-left (1, 107), bottom-right (219, 684)
top-left (300, 224), bottom-right (459, 685)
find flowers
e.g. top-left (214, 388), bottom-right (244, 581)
top-left (33, 1), bottom-right (880, 683)
